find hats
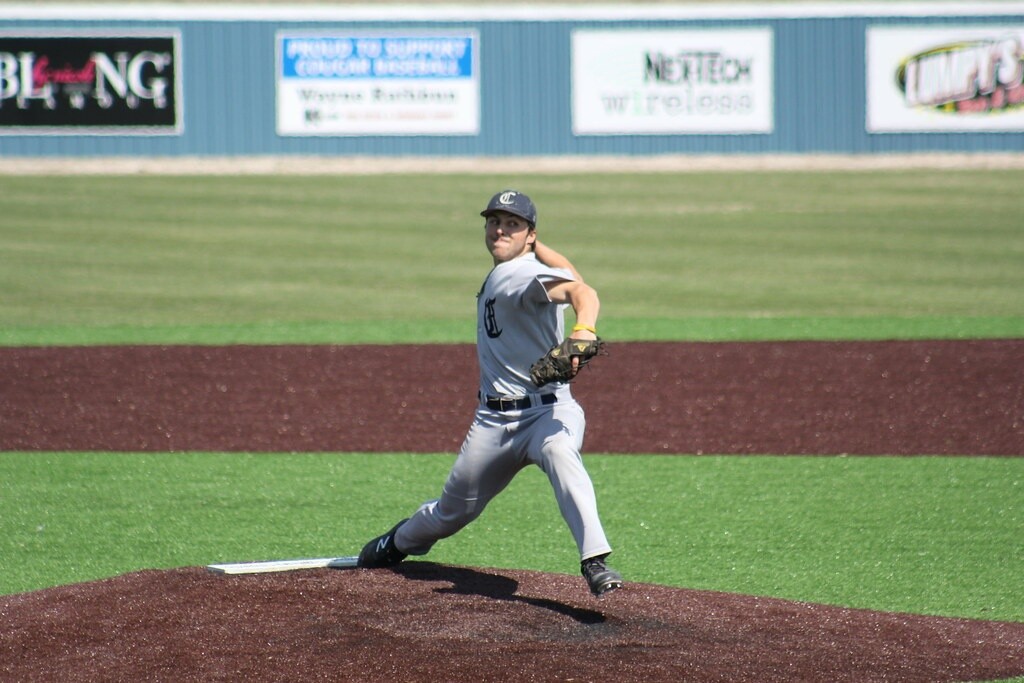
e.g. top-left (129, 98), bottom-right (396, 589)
top-left (480, 190), bottom-right (537, 228)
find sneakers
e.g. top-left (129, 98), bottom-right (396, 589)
top-left (356, 517), bottom-right (412, 569)
top-left (581, 558), bottom-right (623, 600)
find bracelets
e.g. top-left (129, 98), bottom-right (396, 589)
top-left (572, 323), bottom-right (596, 334)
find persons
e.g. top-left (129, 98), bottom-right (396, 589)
top-left (354, 190), bottom-right (623, 598)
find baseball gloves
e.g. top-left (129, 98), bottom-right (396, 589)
top-left (529, 336), bottom-right (608, 386)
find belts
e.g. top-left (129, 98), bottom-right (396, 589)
top-left (478, 390), bottom-right (575, 412)
